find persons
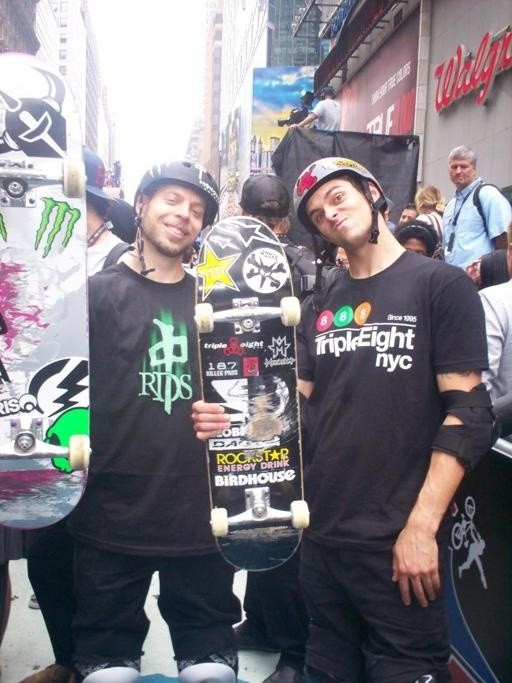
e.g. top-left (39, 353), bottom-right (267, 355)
top-left (288, 85), bottom-right (341, 132)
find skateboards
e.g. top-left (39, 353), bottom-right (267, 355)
top-left (2, 52), bottom-right (90, 531)
top-left (195, 216), bottom-right (311, 570)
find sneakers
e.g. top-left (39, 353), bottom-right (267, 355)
top-left (19, 663), bottom-right (82, 683)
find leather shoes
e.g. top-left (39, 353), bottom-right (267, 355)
top-left (263, 662), bottom-right (303, 683)
top-left (233, 619), bottom-right (280, 653)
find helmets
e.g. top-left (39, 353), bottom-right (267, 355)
top-left (293, 156), bottom-right (389, 234)
top-left (134, 159), bottom-right (220, 230)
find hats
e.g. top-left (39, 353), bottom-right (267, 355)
top-left (238, 175), bottom-right (290, 218)
top-left (83, 144), bottom-right (122, 207)
